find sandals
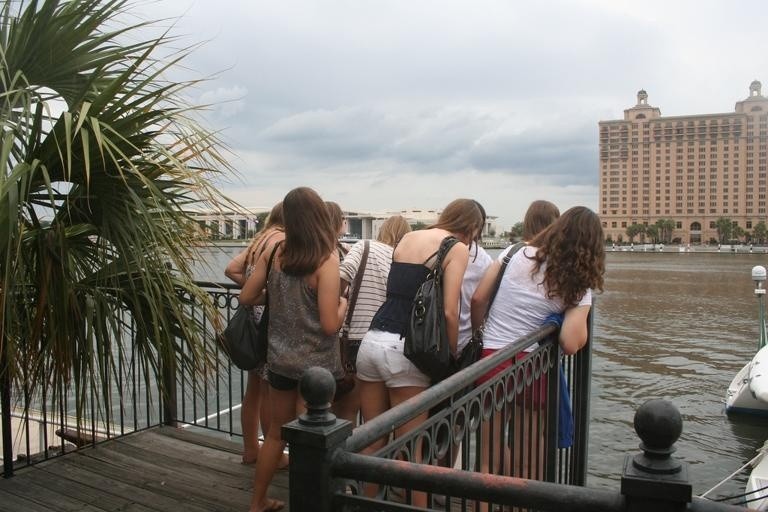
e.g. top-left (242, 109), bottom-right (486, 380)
top-left (266, 500), bottom-right (284, 511)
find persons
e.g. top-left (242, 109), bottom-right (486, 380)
top-left (471, 206), bottom-right (605, 512)
top-left (355, 198), bottom-right (483, 509)
top-left (326, 202), bottom-right (351, 300)
top-left (224, 203), bottom-right (290, 471)
top-left (422, 201), bottom-right (494, 508)
top-left (504, 200), bottom-right (574, 476)
top-left (239, 186), bottom-right (348, 512)
top-left (338, 215), bottom-right (413, 427)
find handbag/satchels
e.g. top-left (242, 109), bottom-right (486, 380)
top-left (405, 277), bottom-right (450, 375)
top-left (330, 336), bottom-right (355, 399)
top-left (449, 336), bottom-right (482, 424)
top-left (223, 305), bottom-right (269, 370)
